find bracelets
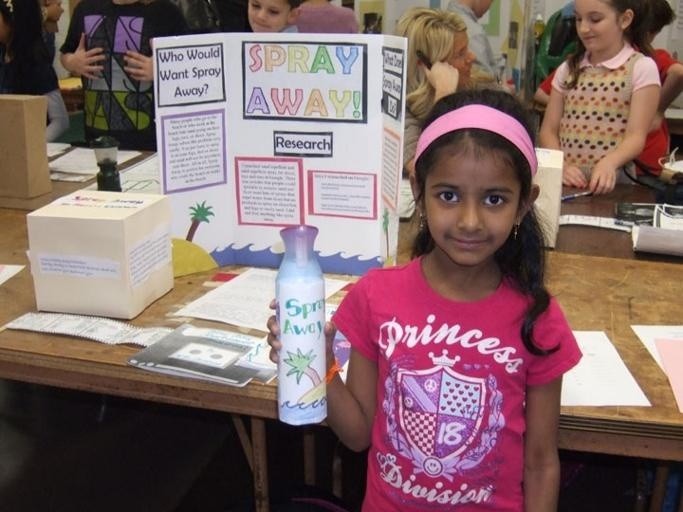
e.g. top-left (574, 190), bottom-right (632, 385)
top-left (326, 358), bottom-right (344, 384)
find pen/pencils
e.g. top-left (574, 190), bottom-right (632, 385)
top-left (615, 221), bottom-right (635, 227)
top-left (561, 192), bottom-right (593, 201)
top-left (416, 50), bottom-right (432, 71)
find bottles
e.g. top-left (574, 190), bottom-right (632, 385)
top-left (275, 225), bottom-right (329, 426)
top-left (506, 79), bottom-right (516, 95)
top-left (93, 136), bottom-right (123, 191)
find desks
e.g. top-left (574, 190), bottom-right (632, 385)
top-left (0, 140), bottom-right (683, 512)
top-left (59, 76), bottom-right (88, 117)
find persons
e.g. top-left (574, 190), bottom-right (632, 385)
top-left (59, 1), bottom-right (191, 152)
top-left (392, 7), bottom-right (502, 173)
top-left (1, 0), bottom-right (68, 144)
top-left (540, 0), bottom-right (662, 197)
top-left (446, 0), bottom-right (505, 80)
top-left (627, 1), bottom-right (683, 176)
top-left (176, 0), bottom-right (361, 33)
top-left (264, 88), bottom-right (584, 511)
